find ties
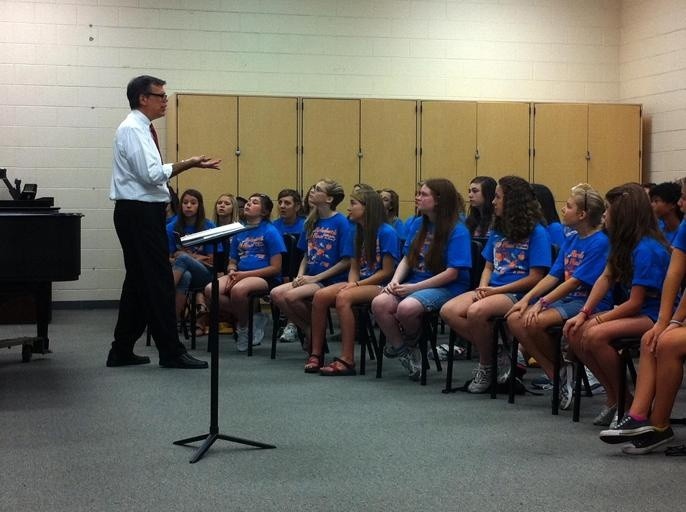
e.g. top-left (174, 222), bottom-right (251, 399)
top-left (150, 123), bottom-right (163, 165)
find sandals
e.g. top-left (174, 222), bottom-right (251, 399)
top-left (320, 357), bottom-right (356, 376)
top-left (304, 354), bottom-right (325, 373)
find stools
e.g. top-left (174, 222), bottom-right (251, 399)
top-left (168, 281), bottom-right (686, 431)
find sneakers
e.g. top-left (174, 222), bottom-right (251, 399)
top-left (252, 315), bottom-right (268, 345)
top-left (593, 395), bottom-right (676, 454)
top-left (384, 327), bottom-right (423, 358)
top-left (398, 349), bottom-right (421, 381)
top-left (236, 322), bottom-right (248, 352)
top-left (468, 340), bottom-right (601, 410)
top-left (279, 323), bottom-right (298, 342)
top-left (428, 343), bottom-right (468, 360)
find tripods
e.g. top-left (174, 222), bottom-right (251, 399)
top-left (171, 285), bottom-right (277, 466)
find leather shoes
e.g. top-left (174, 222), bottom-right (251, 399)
top-left (106, 352), bottom-right (150, 367)
top-left (159, 351), bottom-right (208, 369)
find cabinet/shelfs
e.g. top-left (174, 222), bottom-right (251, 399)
top-left (164, 92), bottom-right (301, 223)
top-left (420, 100), bottom-right (530, 209)
top-left (532, 101), bottom-right (644, 220)
top-left (299, 97), bottom-right (419, 224)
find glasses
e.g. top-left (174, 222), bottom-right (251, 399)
top-left (312, 184), bottom-right (328, 195)
top-left (148, 92), bottom-right (167, 100)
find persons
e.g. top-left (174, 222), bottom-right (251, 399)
top-left (106, 75), bottom-right (220, 369)
top-left (166, 176), bottom-right (685, 456)
